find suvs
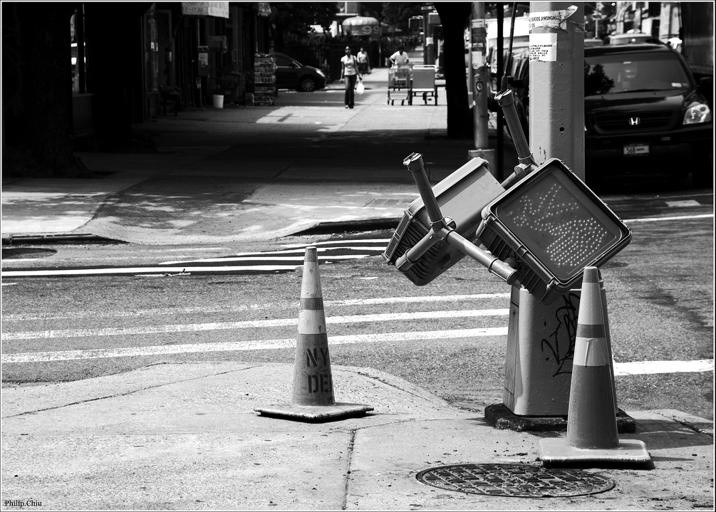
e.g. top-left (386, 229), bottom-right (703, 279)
top-left (505, 41), bottom-right (712, 192)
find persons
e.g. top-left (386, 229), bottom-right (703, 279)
top-left (337, 44), bottom-right (361, 110)
top-left (387, 44), bottom-right (409, 93)
top-left (356, 45), bottom-right (371, 79)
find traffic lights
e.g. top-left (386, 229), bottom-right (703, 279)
top-left (479, 165), bottom-right (635, 291)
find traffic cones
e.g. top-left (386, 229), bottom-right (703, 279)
top-left (533, 265), bottom-right (652, 469)
top-left (254, 245), bottom-right (372, 423)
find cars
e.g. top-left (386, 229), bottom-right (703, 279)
top-left (466, 1), bottom-right (714, 142)
top-left (268, 52), bottom-right (325, 93)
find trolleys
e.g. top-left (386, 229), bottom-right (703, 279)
top-left (385, 62), bottom-right (438, 109)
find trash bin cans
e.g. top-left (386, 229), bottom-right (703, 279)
top-left (424, 44), bottom-right (435, 65)
